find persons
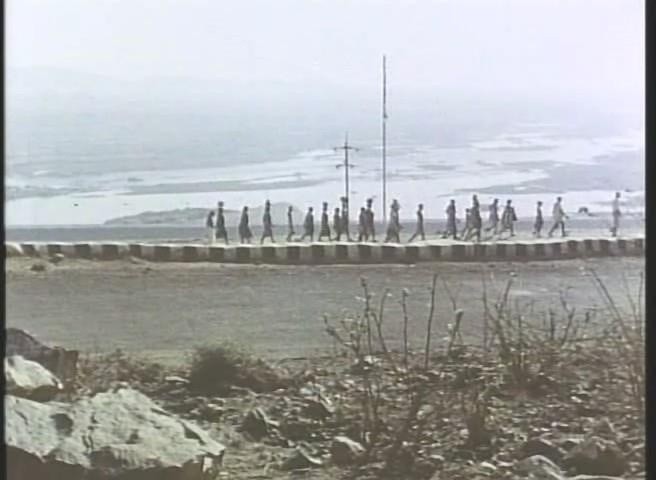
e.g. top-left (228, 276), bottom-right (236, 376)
top-left (408, 204), bottom-right (425, 242)
top-left (442, 189), bottom-right (518, 241)
top-left (384, 200), bottom-right (402, 243)
top-left (238, 206), bottom-right (253, 244)
top-left (535, 194), bottom-right (569, 240)
top-left (206, 197), bottom-right (233, 245)
top-left (608, 191), bottom-right (624, 237)
top-left (286, 202), bottom-right (354, 242)
top-left (260, 199), bottom-right (277, 244)
top-left (358, 199), bottom-right (377, 242)
